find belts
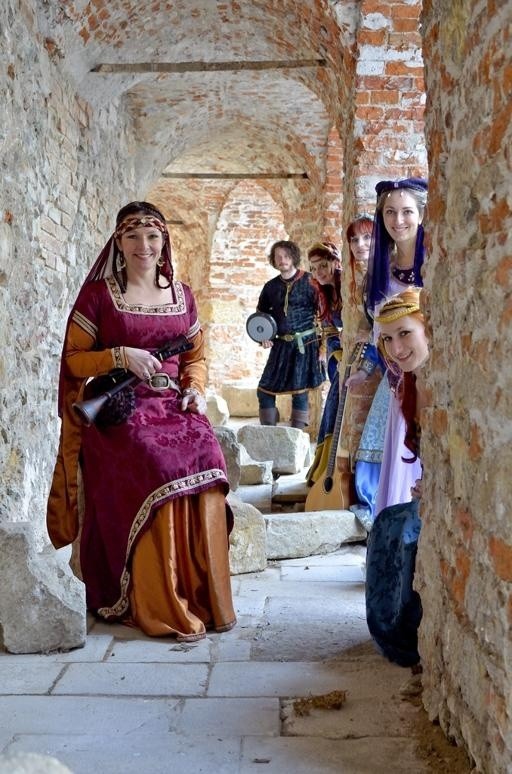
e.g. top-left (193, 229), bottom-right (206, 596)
top-left (141, 371), bottom-right (184, 396)
top-left (275, 326), bottom-right (317, 356)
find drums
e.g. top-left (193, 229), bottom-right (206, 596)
top-left (247, 312), bottom-right (277, 343)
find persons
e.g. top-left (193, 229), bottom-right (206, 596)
top-left (361, 283), bottom-right (431, 696)
top-left (300, 241), bottom-right (344, 486)
top-left (341, 172), bottom-right (430, 535)
top-left (333, 209), bottom-right (384, 463)
top-left (44, 194), bottom-right (239, 645)
top-left (252, 238), bottom-right (325, 432)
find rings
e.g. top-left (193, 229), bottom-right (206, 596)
top-left (143, 372), bottom-right (150, 379)
